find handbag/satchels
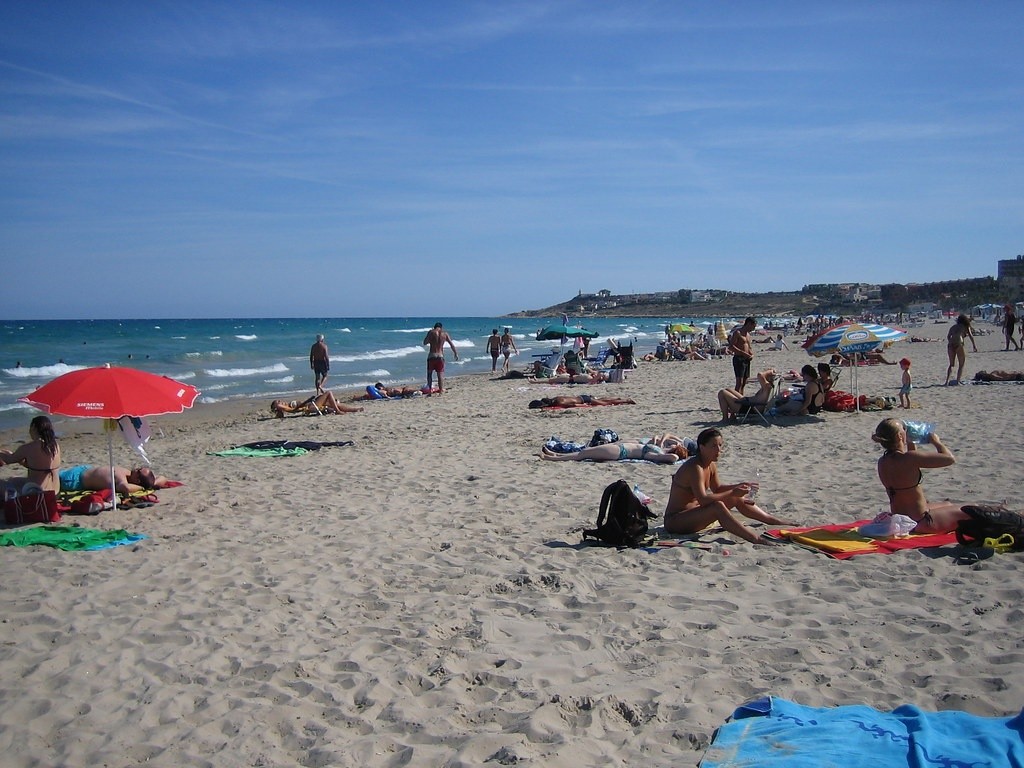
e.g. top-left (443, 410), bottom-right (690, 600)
top-left (858, 511), bottom-right (917, 537)
top-left (2, 485), bottom-right (61, 525)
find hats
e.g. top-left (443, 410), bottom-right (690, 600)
top-left (899, 358), bottom-right (910, 366)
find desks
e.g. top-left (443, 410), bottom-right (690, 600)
top-left (532, 354), bottom-right (552, 362)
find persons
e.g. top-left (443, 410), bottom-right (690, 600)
top-left (831, 342), bottom-right (897, 368)
top-left (531, 433), bottom-right (697, 463)
top-left (911, 304), bottom-right (1024, 387)
top-left (899, 358), bottom-right (912, 409)
top-left (718, 317), bottom-right (834, 424)
top-left (529, 395), bottom-right (636, 408)
top-left (486, 328), bottom-right (519, 375)
top-left (836, 312), bottom-right (925, 324)
top-left (58, 465), bottom-right (169, 494)
top-left (662, 427), bottom-right (805, 547)
top-left (424, 322), bottom-right (458, 397)
top-left (872, 417), bottom-right (1008, 535)
top-left (0, 415), bottom-right (62, 496)
top-left (528, 322), bottom-right (637, 384)
top-left (644, 317), bottom-right (734, 362)
top-left (353, 381), bottom-right (417, 399)
top-left (310, 334), bottom-right (330, 394)
top-left (271, 390), bottom-right (365, 418)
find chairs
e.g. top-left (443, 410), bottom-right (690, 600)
top-left (735, 377), bottom-right (781, 426)
top-left (618, 340), bottom-right (634, 369)
top-left (539, 355), bottom-right (563, 377)
top-left (586, 346), bottom-right (611, 370)
top-left (564, 353), bottom-right (587, 384)
top-left (791, 366), bottom-right (842, 393)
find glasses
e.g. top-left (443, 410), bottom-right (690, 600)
top-left (138, 467), bottom-right (142, 479)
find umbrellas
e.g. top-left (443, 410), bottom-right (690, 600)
top-left (681, 326), bottom-right (706, 335)
top-left (808, 320), bottom-right (910, 414)
top-left (16, 360), bottom-right (201, 510)
top-left (668, 323), bottom-right (694, 333)
top-left (801, 321), bottom-right (907, 395)
top-left (535, 324), bottom-right (599, 356)
top-left (730, 324), bottom-right (743, 333)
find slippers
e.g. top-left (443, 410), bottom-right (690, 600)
top-left (957, 548), bottom-right (995, 563)
top-left (116, 494), bottom-right (160, 509)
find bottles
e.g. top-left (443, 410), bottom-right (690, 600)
top-left (633, 485), bottom-right (643, 504)
top-left (783, 389), bottom-right (787, 397)
top-left (798, 389), bottom-right (801, 394)
top-left (744, 468), bottom-right (759, 505)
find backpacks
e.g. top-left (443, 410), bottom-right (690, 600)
top-left (956, 505), bottom-right (1024, 552)
top-left (596, 482), bottom-right (657, 544)
